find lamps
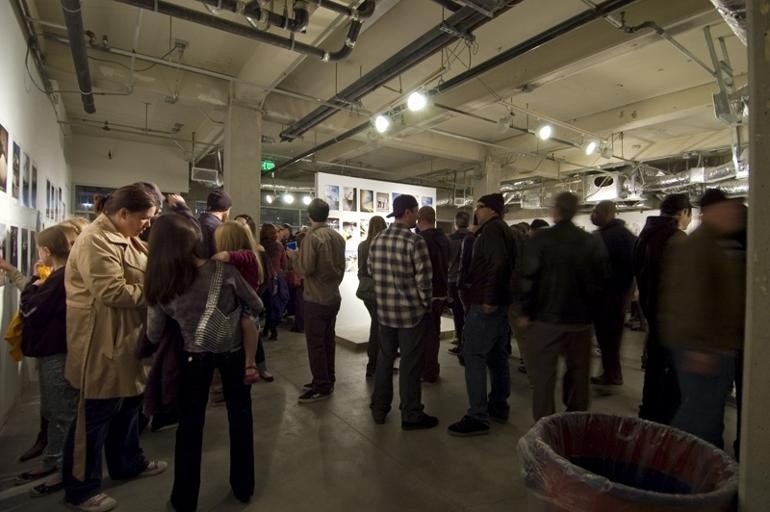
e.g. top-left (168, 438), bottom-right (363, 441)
top-left (712, 92), bottom-right (734, 127)
top-left (535, 123), bottom-right (551, 141)
top-left (495, 112), bottom-right (516, 134)
top-left (407, 92), bottom-right (427, 113)
top-left (580, 142), bottom-right (597, 158)
top-left (376, 116), bottom-right (394, 134)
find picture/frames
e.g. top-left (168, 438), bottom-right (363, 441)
top-left (325, 185), bottom-right (433, 273)
top-left (0, 123), bottom-right (66, 286)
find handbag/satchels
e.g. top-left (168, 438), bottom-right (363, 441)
top-left (196, 306), bottom-right (243, 353)
top-left (356, 276), bottom-right (376, 301)
top-left (266, 276), bottom-right (288, 321)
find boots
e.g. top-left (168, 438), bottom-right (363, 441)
top-left (626, 301), bottom-right (646, 331)
top-left (257, 362), bottom-right (272, 381)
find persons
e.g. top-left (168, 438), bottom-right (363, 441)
top-left (0, 181), bottom-right (306, 511)
top-left (286, 198), bottom-right (345, 404)
top-left (355, 189), bottom-right (750, 463)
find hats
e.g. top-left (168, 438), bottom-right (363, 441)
top-left (478, 193), bottom-right (503, 214)
top-left (207, 191), bottom-right (232, 211)
top-left (386, 194), bottom-right (416, 218)
top-left (661, 194), bottom-right (696, 211)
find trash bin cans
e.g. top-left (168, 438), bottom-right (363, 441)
top-left (517, 410), bottom-right (740, 512)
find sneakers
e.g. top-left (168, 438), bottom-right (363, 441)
top-left (448, 401), bottom-right (508, 436)
top-left (448, 338), bottom-right (458, 354)
top-left (65, 492), bottom-right (116, 512)
top-left (402, 413), bottom-right (439, 430)
top-left (373, 411), bottom-right (385, 423)
top-left (260, 310), bottom-right (303, 341)
top-left (298, 383), bottom-right (334, 403)
top-left (590, 375), bottom-right (622, 385)
top-left (139, 460), bottom-right (167, 476)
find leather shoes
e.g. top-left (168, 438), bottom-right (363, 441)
top-left (245, 367), bottom-right (256, 384)
top-left (15, 463), bottom-right (56, 484)
top-left (19, 443), bottom-right (43, 462)
top-left (31, 481), bottom-right (62, 495)
